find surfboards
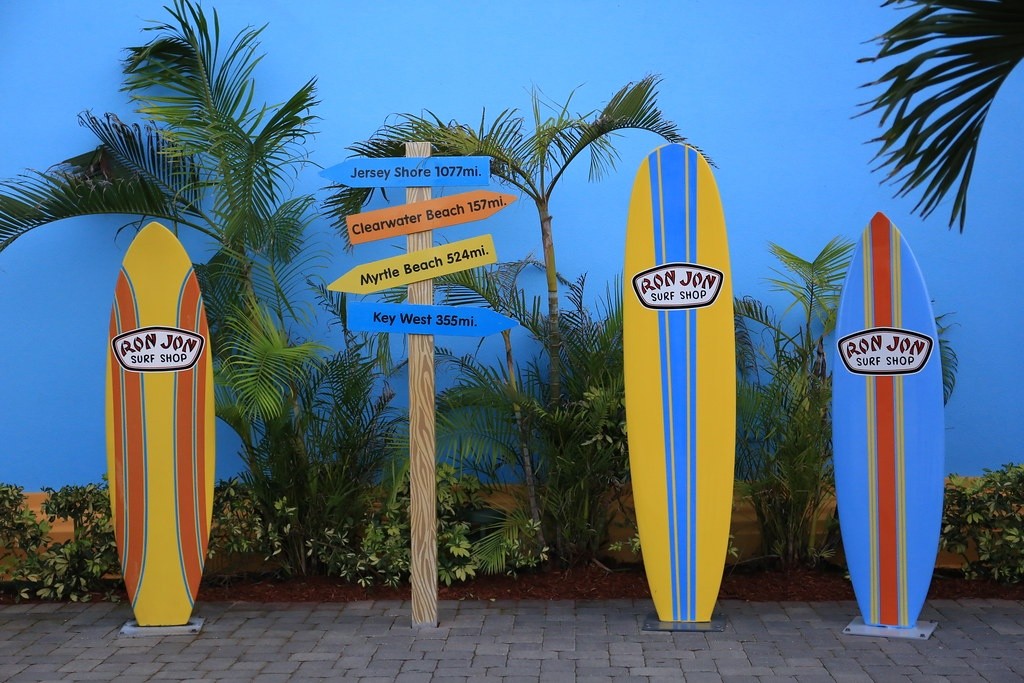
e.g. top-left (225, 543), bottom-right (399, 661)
top-left (832, 209), bottom-right (947, 629)
top-left (620, 143), bottom-right (739, 623)
top-left (103, 221), bottom-right (215, 626)
